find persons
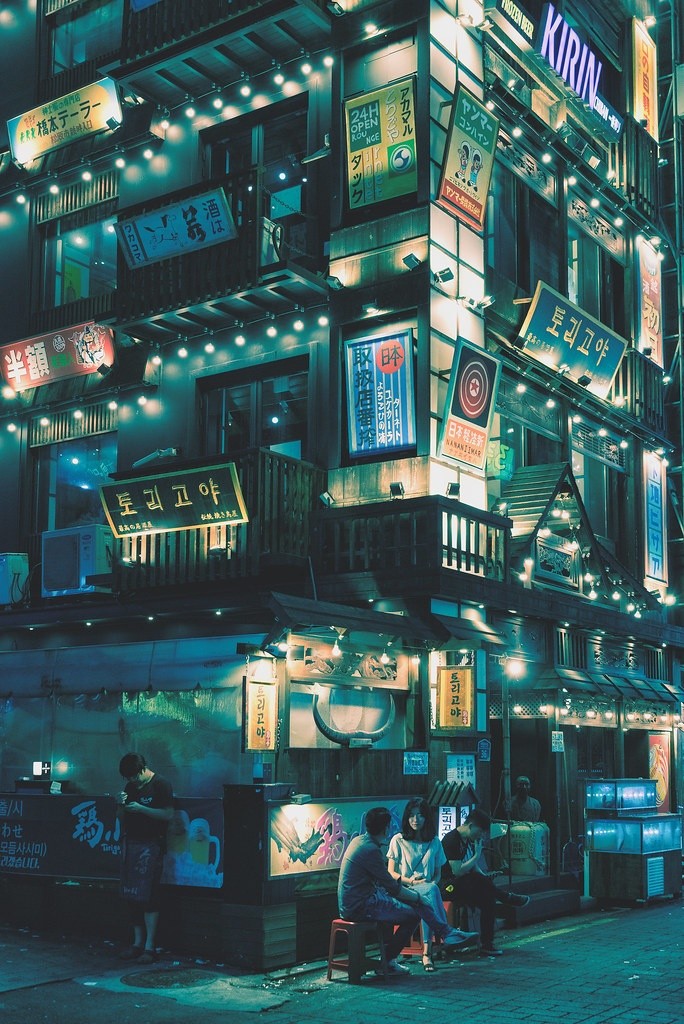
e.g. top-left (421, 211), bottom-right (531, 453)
top-left (502, 776), bottom-right (541, 823)
top-left (440, 809), bottom-right (530, 956)
top-left (114, 752), bottom-right (174, 962)
top-left (337, 808), bottom-right (479, 974)
top-left (384, 797), bottom-right (463, 971)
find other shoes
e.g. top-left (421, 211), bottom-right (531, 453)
top-left (137, 950), bottom-right (161, 964)
top-left (119, 945), bottom-right (145, 960)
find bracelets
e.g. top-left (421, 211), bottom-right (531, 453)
top-left (415, 892), bottom-right (420, 903)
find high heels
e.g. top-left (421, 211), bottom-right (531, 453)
top-left (421, 954), bottom-right (436, 972)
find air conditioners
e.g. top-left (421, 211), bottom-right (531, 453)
top-left (40, 524), bottom-right (116, 599)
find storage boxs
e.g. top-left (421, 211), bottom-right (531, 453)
top-left (584, 777), bottom-right (657, 817)
top-left (587, 813), bottom-right (684, 855)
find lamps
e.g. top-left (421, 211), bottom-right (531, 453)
top-left (511, 76), bottom-right (526, 90)
top-left (326, 253), bottom-right (498, 315)
top-left (327, 2), bottom-right (344, 16)
top-left (445, 483), bottom-right (461, 498)
top-left (656, 158), bottom-right (669, 167)
top-left (639, 119), bottom-right (647, 128)
top-left (642, 347), bottom-right (651, 357)
top-left (513, 336), bottom-right (528, 352)
top-left (390, 481), bottom-right (405, 497)
top-left (266, 645), bottom-right (286, 659)
top-left (97, 363), bottom-right (110, 376)
top-left (289, 792), bottom-right (313, 805)
top-left (319, 491), bottom-right (334, 507)
top-left (106, 117), bottom-right (121, 132)
top-left (208, 544), bottom-right (229, 556)
top-left (490, 496), bottom-right (508, 514)
top-left (0, 552), bottom-right (31, 605)
top-left (457, 11), bottom-right (495, 36)
top-left (117, 555), bottom-right (137, 569)
top-left (576, 376), bottom-right (592, 389)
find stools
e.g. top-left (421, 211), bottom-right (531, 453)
top-left (327, 916), bottom-right (389, 982)
top-left (431, 901), bottom-right (453, 943)
top-left (393, 921), bottom-right (425, 958)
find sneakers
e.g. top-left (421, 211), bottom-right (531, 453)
top-left (443, 929), bottom-right (480, 951)
top-left (502, 892), bottom-right (530, 908)
top-left (375, 959), bottom-right (411, 976)
top-left (480, 944), bottom-right (503, 955)
